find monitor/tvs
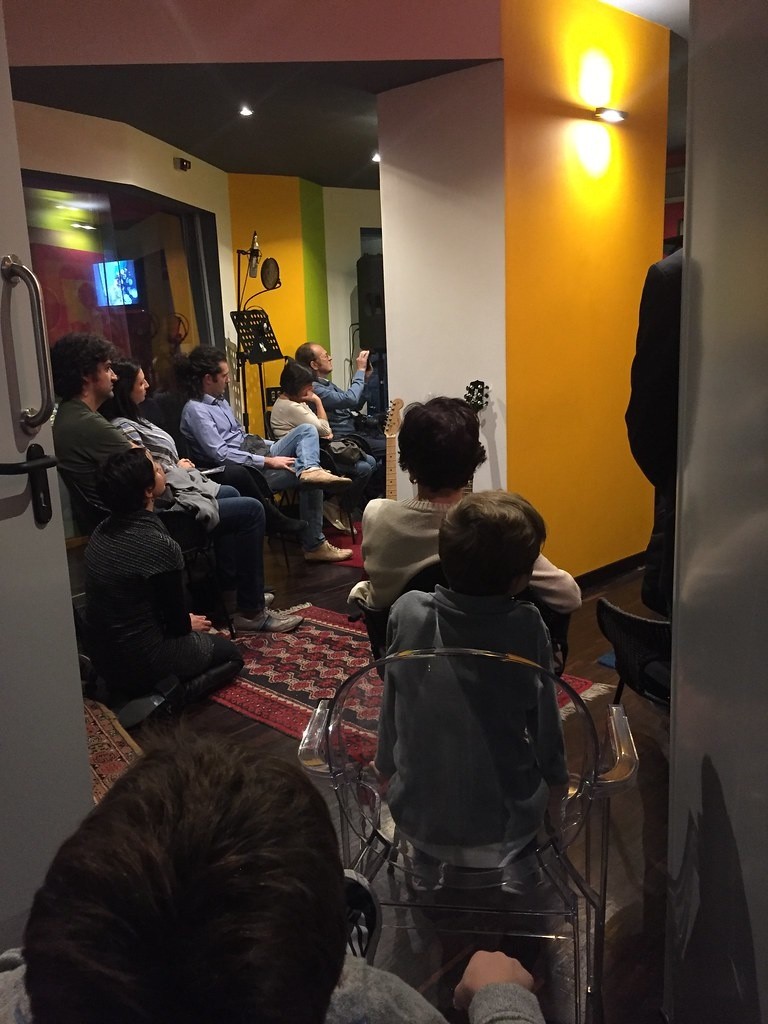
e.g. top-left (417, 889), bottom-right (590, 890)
top-left (92, 258), bottom-right (145, 310)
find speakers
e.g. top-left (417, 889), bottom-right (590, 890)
top-left (357, 252), bottom-right (387, 355)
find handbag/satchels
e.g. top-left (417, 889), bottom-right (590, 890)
top-left (239, 435), bottom-right (270, 456)
top-left (326, 437), bottom-right (365, 467)
top-left (353, 412), bottom-right (383, 437)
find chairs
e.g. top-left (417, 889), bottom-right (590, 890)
top-left (597, 595), bottom-right (671, 756)
top-left (297, 648), bottom-right (639, 1022)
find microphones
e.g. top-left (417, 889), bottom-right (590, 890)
top-left (249, 231), bottom-right (259, 278)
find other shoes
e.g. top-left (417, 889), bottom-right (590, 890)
top-left (117, 672), bottom-right (184, 728)
top-left (412, 857), bottom-right (446, 890)
top-left (503, 866), bottom-right (544, 895)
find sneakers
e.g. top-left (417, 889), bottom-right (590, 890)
top-left (303, 539), bottom-right (354, 562)
top-left (323, 499), bottom-right (357, 536)
top-left (216, 588), bottom-right (275, 620)
top-left (298, 466), bottom-right (353, 491)
top-left (233, 610), bottom-right (304, 632)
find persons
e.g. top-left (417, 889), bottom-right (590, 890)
top-left (360, 397), bottom-right (584, 616)
top-left (374, 492), bottom-right (569, 872)
top-left (50, 333), bottom-right (387, 634)
top-left (625, 244), bottom-right (682, 620)
top-left (0, 736), bottom-right (544, 1024)
top-left (81, 448), bottom-right (244, 729)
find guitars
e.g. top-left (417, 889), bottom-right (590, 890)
top-left (463, 378), bottom-right (492, 501)
top-left (384, 395), bottom-right (406, 502)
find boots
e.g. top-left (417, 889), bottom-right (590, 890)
top-left (263, 497), bottom-right (309, 540)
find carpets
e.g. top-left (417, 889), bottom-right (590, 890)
top-left (84, 695), bottom-right (145, 808)
top-left (204, 602), bottom-right (618, 772)
top-left (321, 518), bottom-right (373, 568)
